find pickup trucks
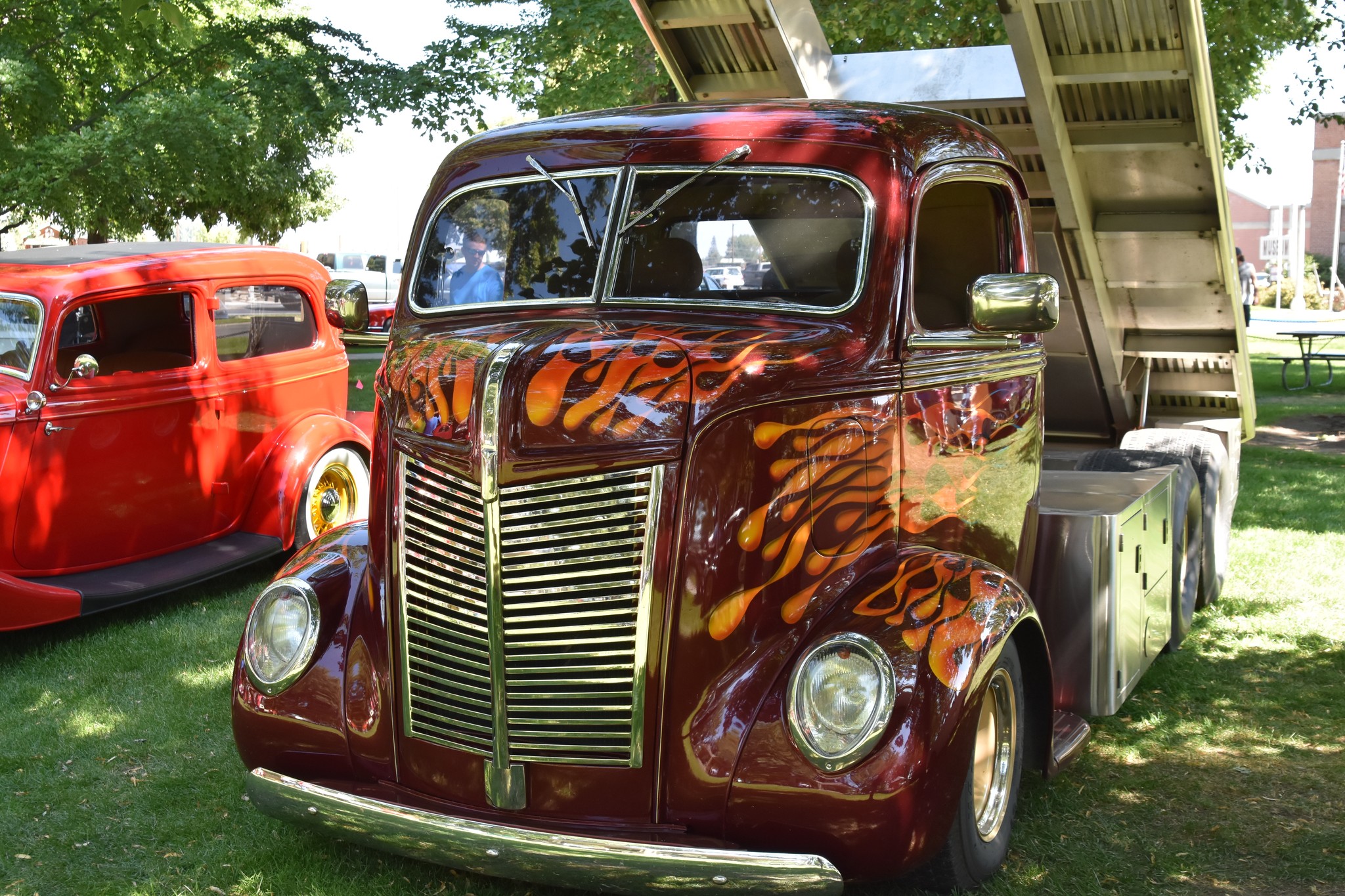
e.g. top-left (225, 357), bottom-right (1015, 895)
top-left (260, 251), bottom-right (404, 311)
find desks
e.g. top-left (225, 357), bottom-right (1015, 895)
top-left (1276, 330), bottom-right (1345, 390)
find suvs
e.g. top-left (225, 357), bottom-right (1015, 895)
top-left (741, 262), bottom-right (772, 287)
top-left (704, 266), bottom-right (745, 290)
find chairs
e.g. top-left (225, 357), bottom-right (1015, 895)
top-left (809, 235), bottom-right (966, 329)
top-left (625, 236), bottom-right (729, 300)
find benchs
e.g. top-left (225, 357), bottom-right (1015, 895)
top-left (1304, 352), bottom-right (1345, 385)
top-left (1264, 355), bottom-right (1345, 392)
top-left (98, 351), bottom-right (191, 375)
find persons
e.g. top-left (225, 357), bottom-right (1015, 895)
top-left (1236, 247), bottom-right (1258, 327)
top-left (449, 227), bottom-right (504, 305)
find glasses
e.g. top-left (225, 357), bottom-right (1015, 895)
top-left (467, 248), bottom-right (485, 255)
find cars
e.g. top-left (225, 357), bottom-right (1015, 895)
top-left (0, 240), bottom-right (375, 631)
top-left (367, 298), bottom-right (397, 333)
top-left (695, 272), bottom-right (721, 291)
top-left (228, 1), bottom-right (1259, 896)
